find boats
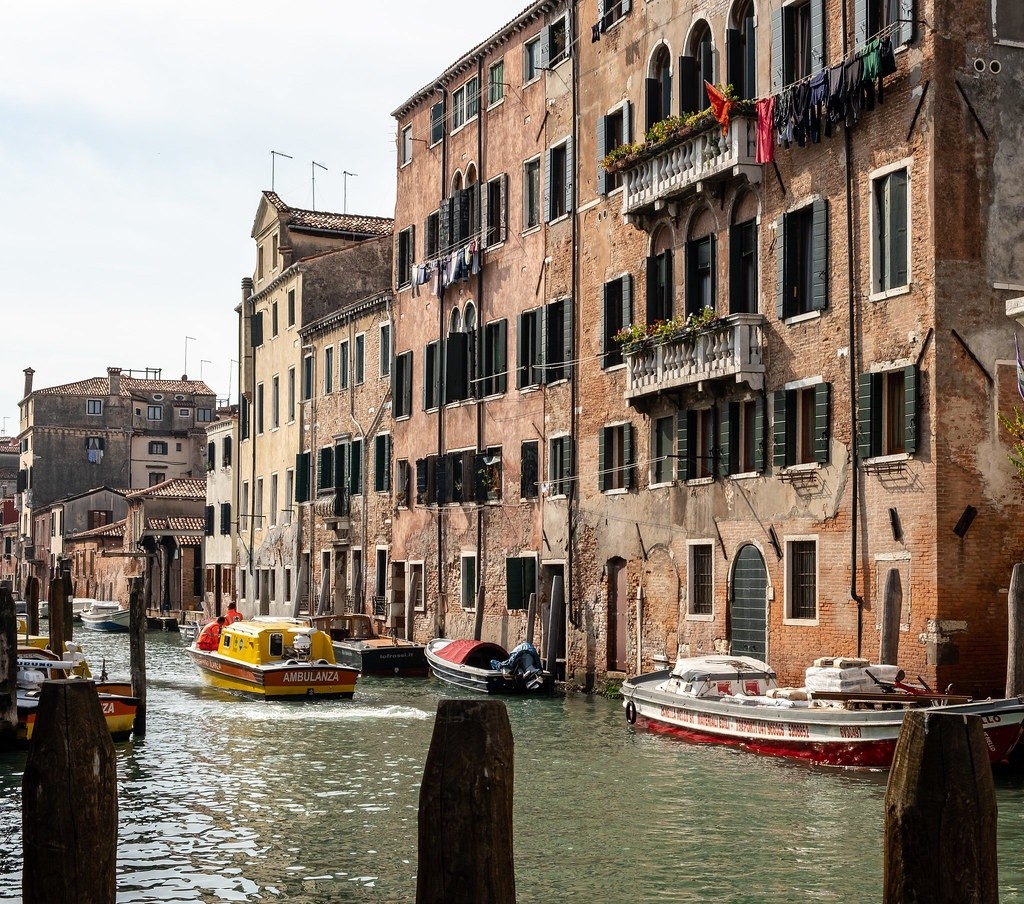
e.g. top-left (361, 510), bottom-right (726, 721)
top-left (9, 589), bottom-right (140, 746)
top-left (185, 616), bottom-right (361, 702)
top-left (618, 653), bottom-right (1024, 774)
top-left (424, 636), bottom-right (553, 695)
top-left (304, 612), bottom-right (430, 678)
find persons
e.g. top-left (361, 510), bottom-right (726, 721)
top-left (223, 602), bottom-right (243, 627)
top-left (197, 617), bottom-right (225, 651)
